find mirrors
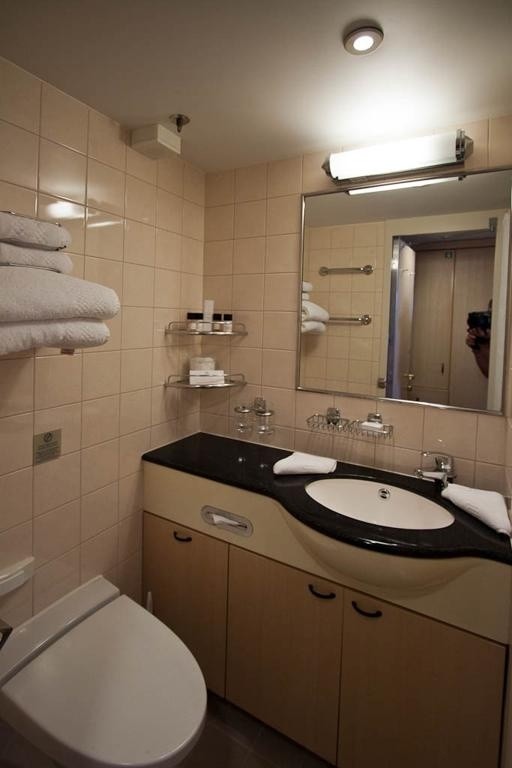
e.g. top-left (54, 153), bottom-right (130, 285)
top-left (292, 168), bottom-right (511, 415)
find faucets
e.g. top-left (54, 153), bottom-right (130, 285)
top-left (415, 450), bottom-right (457, 481)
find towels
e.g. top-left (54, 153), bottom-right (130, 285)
top-left (0, 210), bottom-right (121, 357)
top-left (439, 481), bottom-right (511, 537)
top-left (271, 450), bottom-right (338, 477)
top-left (299, 280), bottom-right (331, 336)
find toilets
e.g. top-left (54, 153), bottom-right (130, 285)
top-left (0, 574), bottom-right (208, 768)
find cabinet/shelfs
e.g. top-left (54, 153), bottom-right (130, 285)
top-left (139, 510), bottom-right (224, 699)
top-left (221, 541), bottom-right (509, 766)
top-left (163, 326), bottom-right (248, 388)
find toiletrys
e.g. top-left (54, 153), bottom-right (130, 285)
top-left (187, 298), bottom-right (235, 333)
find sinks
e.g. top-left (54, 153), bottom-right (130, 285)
top-left (303, 472), bottom-right (455, 531)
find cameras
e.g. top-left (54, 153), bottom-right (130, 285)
top-left (466, 310), bottom-right (492, 326)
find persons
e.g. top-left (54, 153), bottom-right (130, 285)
top-left (464, 298), bottom-right (492, 379)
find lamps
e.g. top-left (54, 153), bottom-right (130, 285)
top-left (321, 128), bottom-right (474, 186)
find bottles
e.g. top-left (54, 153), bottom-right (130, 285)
top-left (211, 312), bottom-right (234, 332)
top-left (186, 312), bottom-right (204, 332)
